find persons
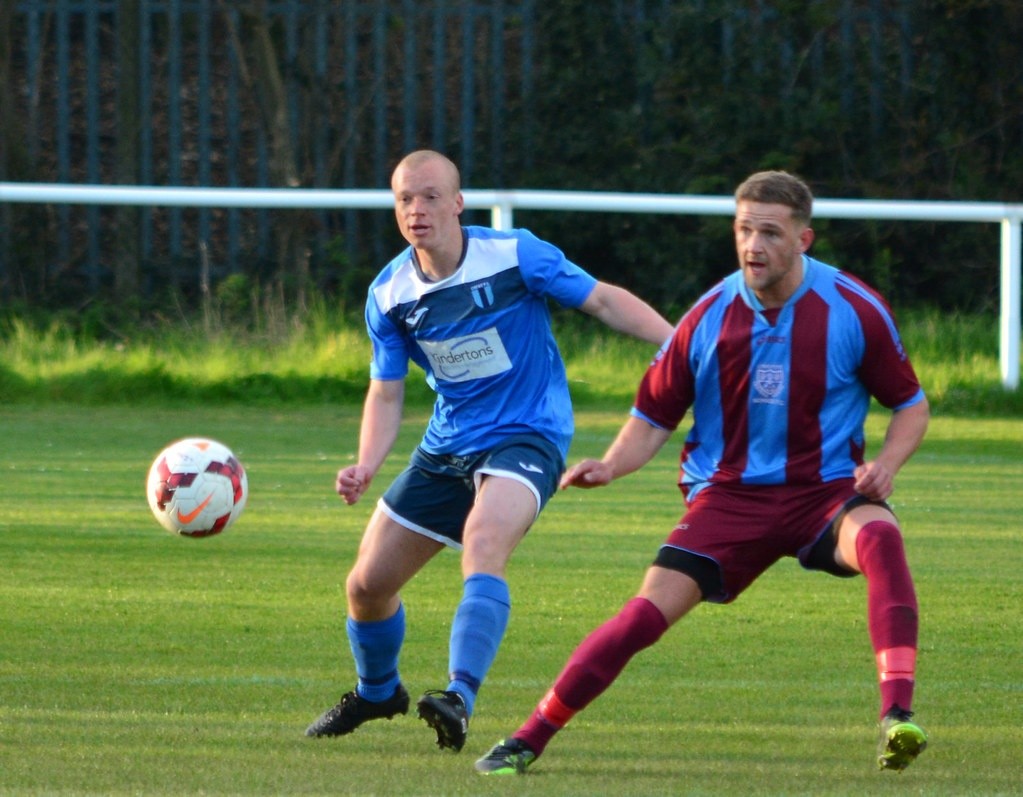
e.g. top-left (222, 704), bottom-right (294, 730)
top-left (472, 170), bottom-right (929, 769)
top-left (304, 148), bottom-right (673, 749)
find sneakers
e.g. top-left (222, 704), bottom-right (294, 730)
top-left (473, 738), bottom-right (536, 777)
top-left (876, 715), bottom-right (928, 775)
top-left (417, 688), bottom-right (470, 754)
top-left (304, 682), bottom-right (410, 739)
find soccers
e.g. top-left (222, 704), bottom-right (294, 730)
top-left (141, 436), bottom-right (250, 542)
